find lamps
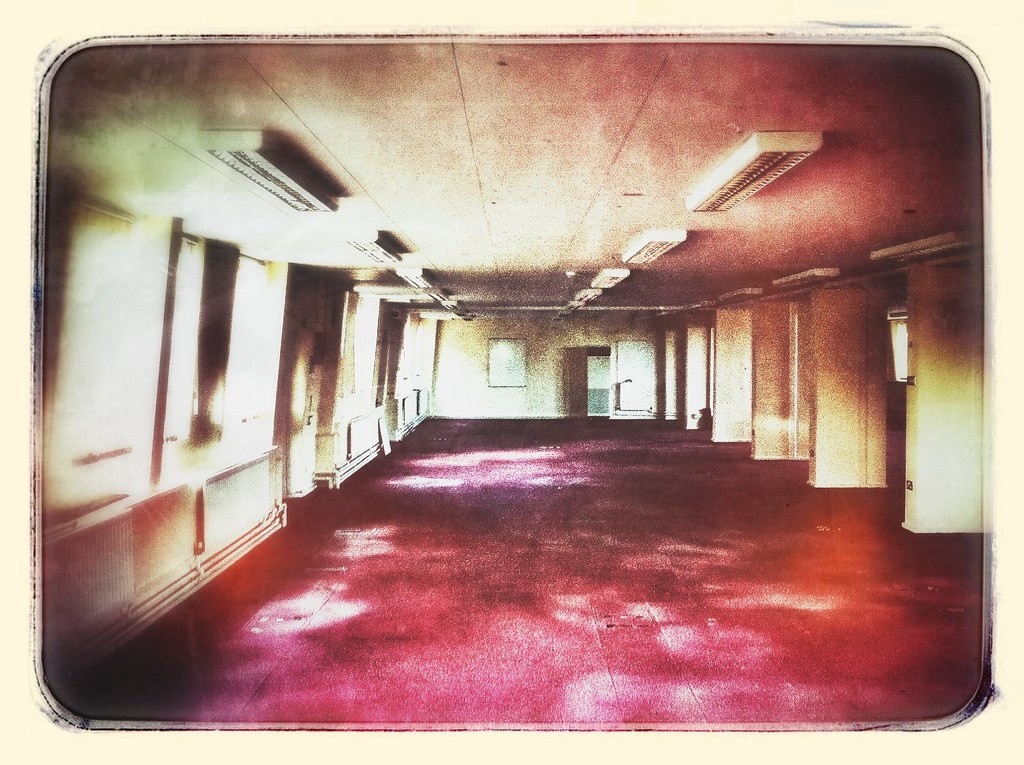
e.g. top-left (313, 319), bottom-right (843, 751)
top-left (688, 132), bottom-right (822, 213)
top-left (426, 290), bottom-right (474, 321)
top-left (395, 268), bottom-right (435, 289)
top-left (621, 229), bottom-right (687, 264)
top-left (343, 230), bottom-right (403, 265)
top-left (565, 269), bottom-right (630, 308)
top-left (191, 130), bottom-right (339, 212)
